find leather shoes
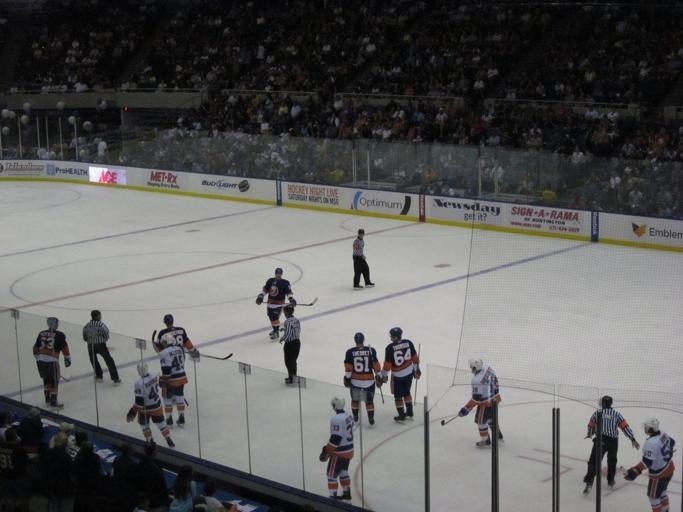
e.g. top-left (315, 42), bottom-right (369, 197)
top-left (354, 285), bottom-right (363, 287)
top-left (364, 281), bottom-right (375, 285)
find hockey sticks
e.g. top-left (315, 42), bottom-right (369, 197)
top-left (185, 351), bottom-right (232, 361)
top-left (61, 374), bottom-right (73, 382)
top-left (152, 330), bottom-right (165, 349)
top-left (263, 297), bottom-right (318, 306)
top-left (368, 344), bottom-right (385, 405)
top-left (620, 449), bottom-right (677, 476)
top-left (441, 415), bottom-right (458, 426)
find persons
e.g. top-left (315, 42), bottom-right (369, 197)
top-left (81, 310), bottom-right (126, 387)
top-left (30, 315), bottom-right (71, 409)
top-left (0, 406), bottom-right (238, 508)
top-left (350, 228), bottom-right (375, 290)
top-left (457, 356), bottom-right (505, 449)
top-left (153, 337), bottom-right (188, 428)
top-left (581, 395), bottom-right (639, 496)
top-left (254, 266), bottom-right (296, 342)
top-left (624, 416), bottom-right (678, 511)
top-left (0, 1), bottom-right (406, 184)
top-left (340, 332), bottom-right (381, 427)
top-left (122, 361), bottom-right (176, 449)
top-left (318, 392), bottom-right (358, 502)
top-left (152, 315), bottom-right (200, 364)
top-left (381, 326), bottom-right (424, 426)
top-left (395, 1), bottom-right (683, 221)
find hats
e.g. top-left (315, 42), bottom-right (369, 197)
top-left (54, 432), bottom-right (68, 448)
top-left (58, 422), bottom-right (74, 433)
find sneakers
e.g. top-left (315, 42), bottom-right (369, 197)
top-left (476, 434), bottom-right (490, 446)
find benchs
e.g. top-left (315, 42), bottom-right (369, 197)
top-left (1, 106), bottom-right (313, 187)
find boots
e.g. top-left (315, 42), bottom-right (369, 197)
top-left (44, 389), bottom-right (51, 403)
top-left (145, 412), bottom-right (184, 450)
top-left (337, 487), bottom-right (351, 499)
top-left (285, 376), bottom-right (292, 384)
top-left (269, 326), bottom-right (279, 339)
top-left (352, 402), bottom-right (413, 426)
top-left (50, 394), bottom-right (64, 407)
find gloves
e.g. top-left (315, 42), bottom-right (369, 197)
top-left (344, 376), bottom-right (351, 387)
top-left (256, 297), bottom-right (263, 305)
top-left (127, 408), bottom-right (137, 422)
top-left (288, 297), bottom-right (296, 306)
top-left (458, 407), bottom-right (468, 417)
top-left (64, 355), bottom-right (71, 367)
top-left (319, 445), bottom-right (329, 462)
top-left (624, 466), bottom-right (641, 481)
top-left (412, 368), bottom-right (421, 379)
top-left (189, 348), bottom-right (199, 358)
top-left (375, 375), bottom-right (388, 388)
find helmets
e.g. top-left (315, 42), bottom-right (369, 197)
top-left (136, 363), bottom-right (149, 377)
top-left (354, 333), bottom-right (363, 344)
top-left (283, 304), bottom-right (294, 317)
top-left (644, 418), bottom-right (659, 434)
top-left (602, 396), bottom-right (612, 408)
top-left (470, 359), bottom-right (482, 372)
top-left (163, 314), bottom-right (173, 324)
top-left (47, 317), bottom-right (58, 328)
top-left (91, 310), bottom-right (101, 321)
top-left (390, 327), bottom-right (402, 340)
top-left (275, 268), bottom-right (282, 274)
top-left (160, 333), bottom-right (174, 345)
top-left (331, 396), bottom-right (346, 410)
